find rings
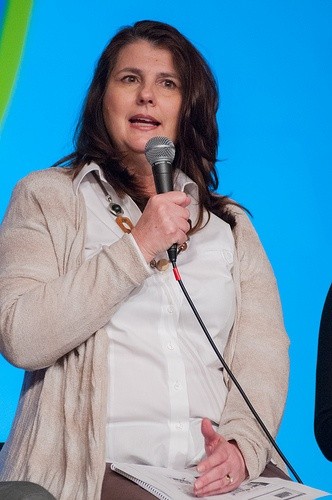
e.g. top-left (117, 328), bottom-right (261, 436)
top-left (226, 475), bottom-right (232, 486)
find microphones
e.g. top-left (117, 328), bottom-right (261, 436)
top-left (144, 136), bottom-right (178, 261)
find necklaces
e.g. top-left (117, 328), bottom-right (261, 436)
top-left (92, 173), bottom-right (191, 273)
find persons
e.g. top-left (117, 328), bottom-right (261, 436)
top-left (0, 20), bottom-right (289, 500)
top-left (313, 285), bottom-right (331, 462)
